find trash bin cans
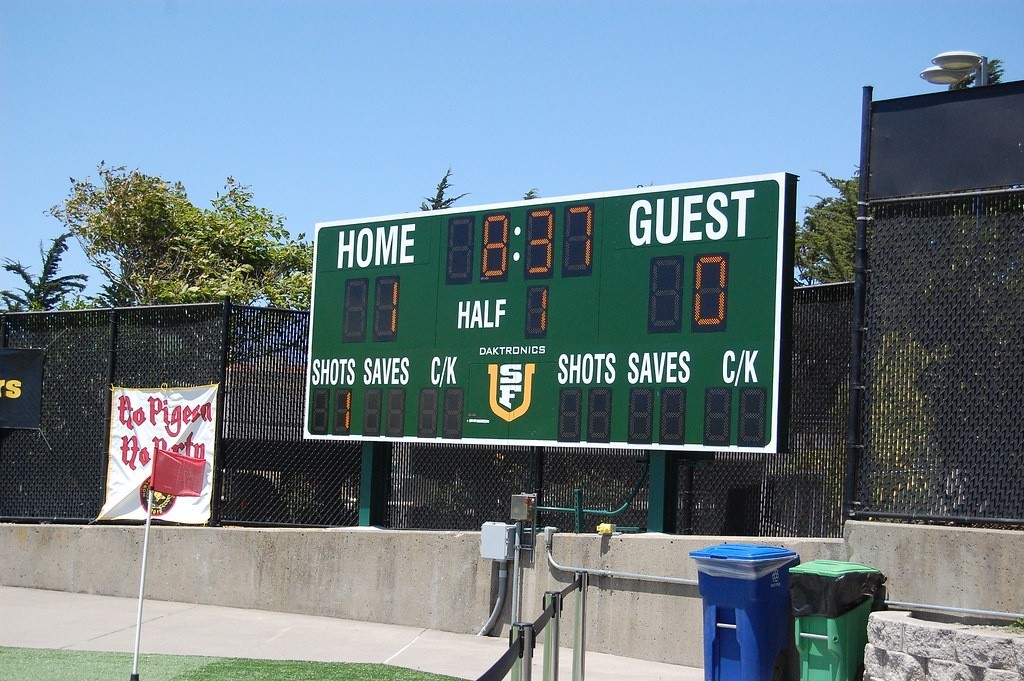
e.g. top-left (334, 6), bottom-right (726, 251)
top-left (789, 558), bottom-right (886, 681)
top-left (688, 543), bottom-right (800, 681)
